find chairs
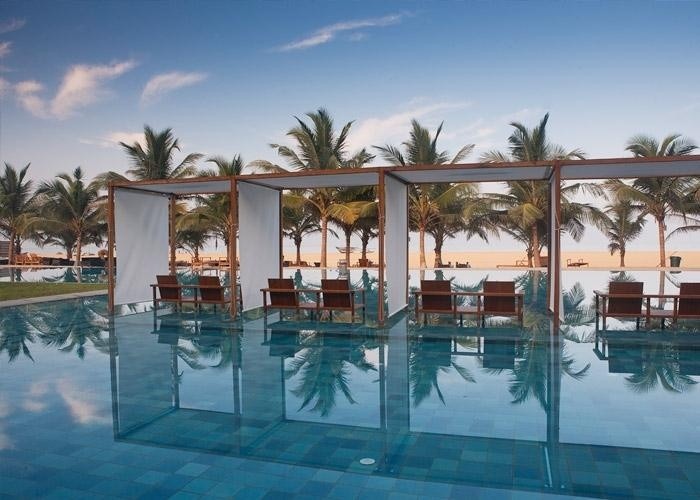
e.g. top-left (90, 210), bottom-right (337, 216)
top-left (337, 258), bottom-right (347, 267)
top-left (14, 252), bottom-right (40, 264)
top-left (579, 259), bottom-right (589, 267)
top-left (192, 256), bottom-right (240, 276)
top-left (567, 259), bottom-right (580, 268)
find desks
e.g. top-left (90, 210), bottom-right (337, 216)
top-left (572, 263), bottom-right (589, 268)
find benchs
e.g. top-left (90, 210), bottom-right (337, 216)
top-left (150, 275), bottom-right (241, 312)
top-left (415, 280), bottom-right (523, 327)
top-left (593, 281), bottom-right (700, 330)
top-left (151, 309), bottom-right (225, 346)
top-left (593, 331), bottom-right (700, 375)
top-left (262, 321), bottom-right (368, 361)
top-left (411, 328), bottom-right (523, 368)
top-left (260, 278), bottom-right (366, 322)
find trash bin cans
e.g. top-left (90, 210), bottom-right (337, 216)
top-left (337, 259), bottom-right (347, 275)
top-left (670, 256), bottom-right (681, 273)
